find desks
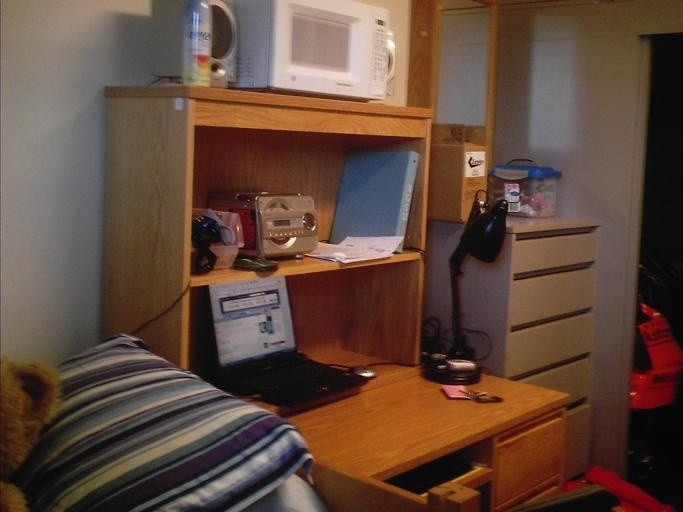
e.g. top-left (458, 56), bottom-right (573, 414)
top-left (240, 355), bottom-right (574, 512)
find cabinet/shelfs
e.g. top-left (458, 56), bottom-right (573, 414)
top-left (423, 208), bottom-right (603, 486)
top-left (94, 83), bottom-right (436, 397)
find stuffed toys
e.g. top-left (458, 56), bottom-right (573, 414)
top-left (0, 355), bottom-right (62, 512)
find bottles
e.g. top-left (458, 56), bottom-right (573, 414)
top-left (181, 1), bottom-right (214, 88)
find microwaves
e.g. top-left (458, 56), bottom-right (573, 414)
top-left (233, 0), bottom-right (399, 102)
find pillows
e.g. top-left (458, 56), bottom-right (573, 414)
top-left (19, 332), bottom-right (313, 510)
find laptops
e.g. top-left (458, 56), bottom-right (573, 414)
top-left (204, 277), bottom-right (366, 409)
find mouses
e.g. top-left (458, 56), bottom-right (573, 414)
top-left (349, 365), bottom-right (377, 379)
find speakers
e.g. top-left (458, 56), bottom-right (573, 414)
top-left (388, 28), bottom-right (397, 98)
top-left (208, 0), bottom-right (237, 88)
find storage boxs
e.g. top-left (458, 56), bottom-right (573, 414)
top-left (426, 121), bottom-right (489, 224)
top-left (491, 160), bottom-right (562, 217)
